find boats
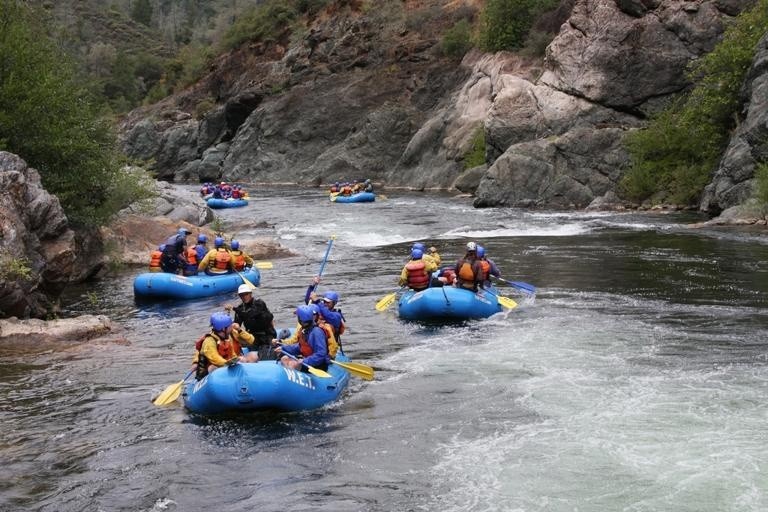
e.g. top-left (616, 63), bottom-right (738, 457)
top-left (132, 261), bottom-right (263, 308)
top-left (395, 275), bottom-right (504, 328)
top-left (205, 197), bottom-right (247, 209)
top-left (181, 327), bottom-right (352, 415)
top-left (202, 192), bottom-right (213, 201)
top-left (327, 191), bottom-right (375, 203)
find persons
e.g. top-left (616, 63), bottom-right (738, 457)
top-left (199, 182), bottom-right (246, 201)
top-left (149, 227), bottom-right (252, 275)
top-left (330, 178), bottom-right (372, 196)
top-left (194, 283), bottom-right (345, 385)
top-left (400, 241), bottom-right (500, 292)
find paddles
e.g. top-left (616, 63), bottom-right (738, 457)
top-left (153, 370), bottom-right (193, 406)
top-left (234, 268), bottom-right (256, 290)
top-left (487, 287), bottom-right (517, 309)
top-left (491, 275), bottom-right (536, 295)
top-left (252, 262), bottom-right (273, 269)
top-left (282, 350), bottom-right (333, 379)
top-left (329, 360), bottom-right (375, 382)
top-left (374, 283), bottom-right (404, 313)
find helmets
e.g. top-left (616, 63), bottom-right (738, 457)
top-left (413, 243), bottom-right (425, 251)
top-left (334, 179), bottom-right (371, 187)
top-left (215, 237), bottom-right (224, 246)
top-left (204, 182), bottom-right (241, 191)
top-left (295, 305), bottom-right (313, 324)
top-left (198, 234), bottom-right (207, 243)
top-left (231, 240), bottom-right (239, 249)
top-left (412, 249), bottom-right (423, 259)
top-left (210, 311), bottom-right (232, 330)
top-left (309, 303), bottom-right (320, 315)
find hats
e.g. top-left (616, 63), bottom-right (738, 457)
top-left (477, 245), bottom-right (485, 257)
top-left (322, 291), bottom-right (338, 303)
top-left (467, 242), bottom-right (477, 251)
top-left (237, 284), bottom-right (252, 294)
top-left (177, 227), bottom-right (192, 237)
